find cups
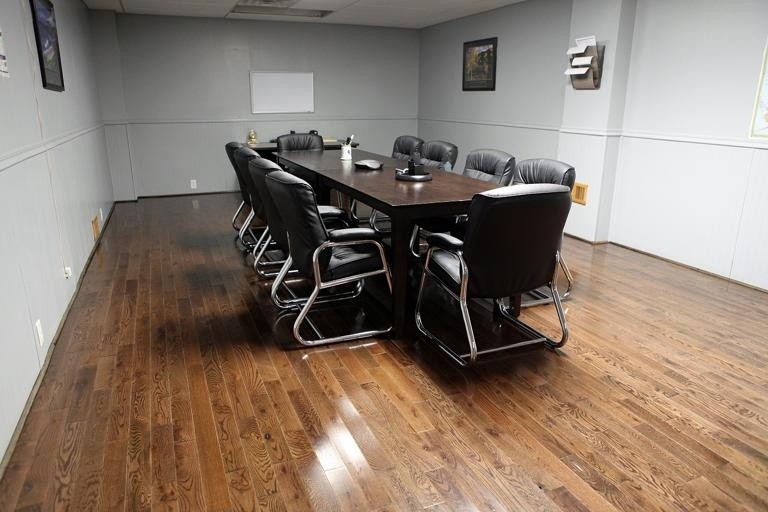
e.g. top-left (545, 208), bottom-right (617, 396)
top-left (340, 146), bottom-right (352, 161)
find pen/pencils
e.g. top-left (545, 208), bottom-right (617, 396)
top-left (346, 134), bottom-right (354, 145)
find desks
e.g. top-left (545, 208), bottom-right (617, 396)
top-left (271, 148), bottom-right (504, 341)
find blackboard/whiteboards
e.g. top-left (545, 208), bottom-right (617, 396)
top-left (250, 71), bottom-right (314, 113)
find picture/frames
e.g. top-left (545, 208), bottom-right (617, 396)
top-left (462, 37), bottom-right (496, 90)
top-left (30, 0), bottom-right (66, 90)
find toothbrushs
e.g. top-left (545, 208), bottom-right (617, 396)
top-left (349, 135), bottom-right (354, 145)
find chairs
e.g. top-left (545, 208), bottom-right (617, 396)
top-left (462, 148), bottom-right (576, 310)
top-left (391, 135), bottom-right (459, 172)
top-left (225, 132), bottom-right (403, 349)
top-left (408, 183), bottom-right (572, 369)
